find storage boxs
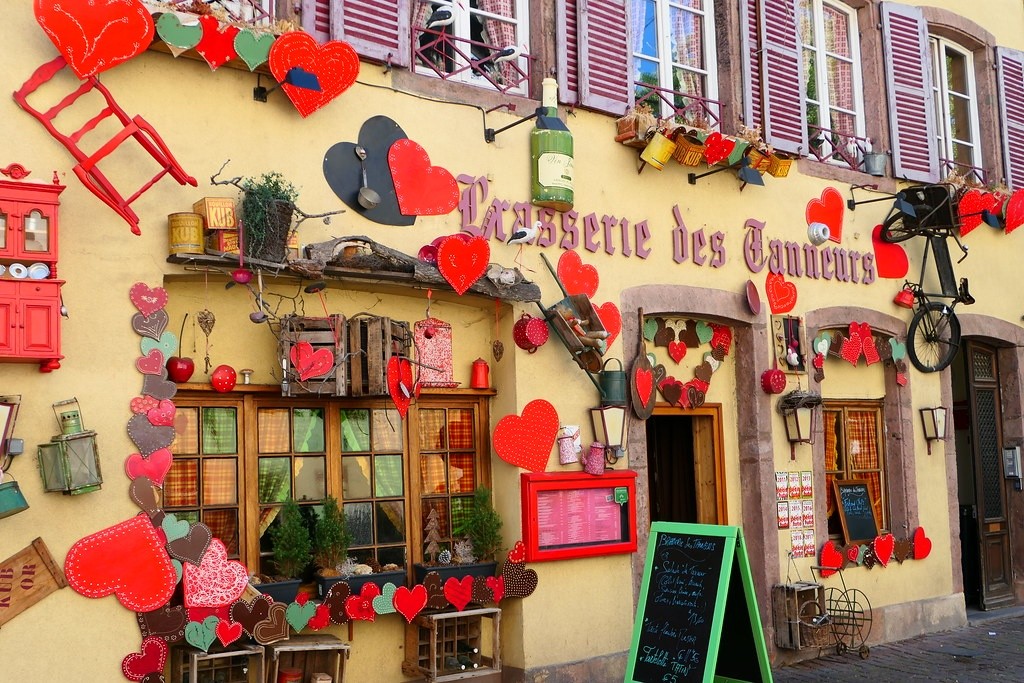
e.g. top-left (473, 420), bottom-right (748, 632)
top-left (745, 146), bottom-right (772, 176)
top-left (672, 133), bottom-right (707, 168)
top-left (771, 578), bottom-right (826, 651)
top-left (209, 230), bottom-right (239, 254)
top-left (401, 608), bottom-right (503, 683)
top-left (170, 640), bottom-right (266, 683)
top-left (264, 634), bottom-right (351, 683)
top-left (192, 196), bottom-right (237, 234)
top-left (277, 314), bottom-right (412, 399)
top-left (767, 152), bottom-right (793, 179)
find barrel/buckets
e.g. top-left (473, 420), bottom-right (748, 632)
top-left (168, 213), bottom-right (204, 255)
top-left (863, 154), bottom-right (888, 177)
top-left (557, 428), bottom-right (578, 465)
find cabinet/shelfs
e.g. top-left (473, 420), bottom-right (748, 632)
top-left (0, 170), bottom-right (66, 279)
top-left (0, 278), bottom-right (66, 373)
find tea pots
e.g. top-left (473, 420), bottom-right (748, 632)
top-left (580, 441), bottom-right (614, 475)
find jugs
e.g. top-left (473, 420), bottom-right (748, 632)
top-left (470, 358), bottom-right (489, 390)
top-left (584, 359), bottom-right (625, 405)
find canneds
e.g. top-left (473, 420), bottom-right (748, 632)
top-left (167, 211), bottom-right (204, 255)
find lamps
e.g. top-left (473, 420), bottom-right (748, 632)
top-left (919, 406), bottom-right (949, 456)
top-left (588, 405), bottom-right (628, 458)
top-left (780, 404), bottom-right (813, 460)
top-left (38, 396), bottom-right (104, 497)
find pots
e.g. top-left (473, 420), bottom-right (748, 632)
top-left (514, 313), bottom-right (549, 354)
top-left (356, 148), bottom-right (381, 209)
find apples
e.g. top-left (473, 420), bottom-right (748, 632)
top-left (165, 355), bottom-right (195, 384)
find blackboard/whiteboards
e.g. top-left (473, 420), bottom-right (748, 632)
top-left (832, 479), bottom-right (882, 546)
top-left (622, 520), bottom-right (774, 683)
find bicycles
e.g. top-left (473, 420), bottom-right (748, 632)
top-left (880, 158), bottom-right (992, 374)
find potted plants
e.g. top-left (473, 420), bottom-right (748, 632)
top-left (253, 497), bottom-right (313, 604)
top-left (412, 481), bottom-right (510, 586)
top-left (312, 494), bottom-right (407, 601)
top-left (237, 170), bottom-right (303, 275)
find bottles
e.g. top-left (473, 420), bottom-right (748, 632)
top-left (439, 641), bottom-right (478, 670)
